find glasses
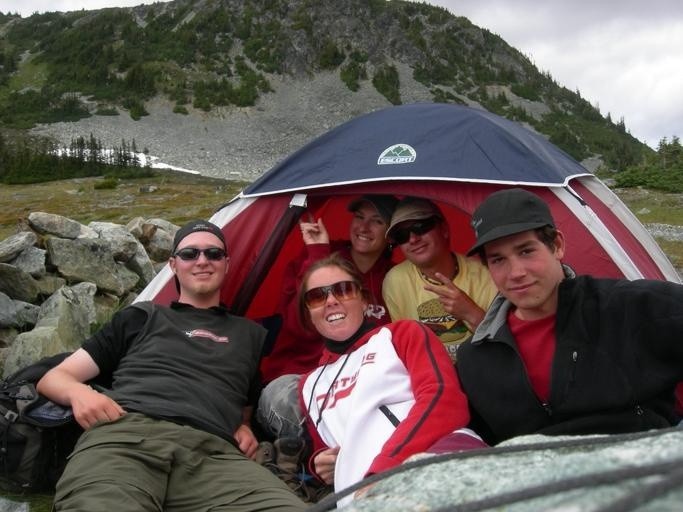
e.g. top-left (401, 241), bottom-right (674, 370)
top-left (393, 219), bottom-right (435, 246)
top-left (303, 280), bottom-right (361, 308)
top-left (175, 247), bottom-right (227, 262)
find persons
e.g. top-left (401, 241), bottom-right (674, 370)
top-left (455, 189), bottom-right (683, 448)
top-left (37, 219), bottom-right (313, 512)
top-left (383, 196), bottom-right (497, 368)
top-left (266, 194), bottom-right (396, 377)
top-left (299, 258), bottom-right (494, 511)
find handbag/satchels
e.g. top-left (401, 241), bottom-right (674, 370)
top-left (0, 352), bottom-right (83, 495)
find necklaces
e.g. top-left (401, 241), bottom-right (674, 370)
top-left (421, 261), bottom-right (458, 286)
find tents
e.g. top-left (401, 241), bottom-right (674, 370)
top-left (132, 104), bottom-right (683, 303)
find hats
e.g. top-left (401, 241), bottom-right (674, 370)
top-left (348, 193), bottom-right (400, 223)
top-left (383, 198), bottom-right (435, 240)
top-left (465, 189), bottom-right (552, 256)
top-left (172, 219), bottom-right (225, 253)
